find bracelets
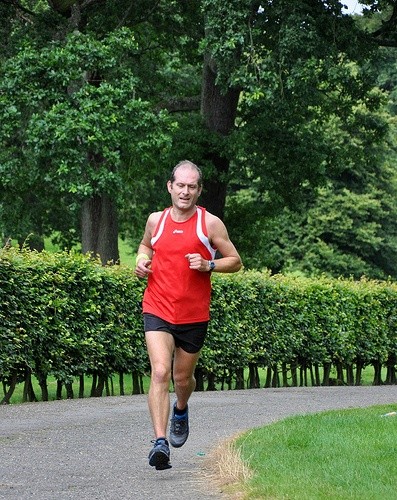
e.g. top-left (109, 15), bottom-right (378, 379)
top-left (137, 254), bottom-right (149, 263)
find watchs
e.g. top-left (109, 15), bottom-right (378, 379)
top-left (208, 260), bottom-right (215, 273)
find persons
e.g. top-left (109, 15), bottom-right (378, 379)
top-left (136, 160), bottom-right (241, 468)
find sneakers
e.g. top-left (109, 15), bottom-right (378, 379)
top-left (169, 401), bottom-right (189, 448)
top-left (147, 439), bottom-right (172, 470)
